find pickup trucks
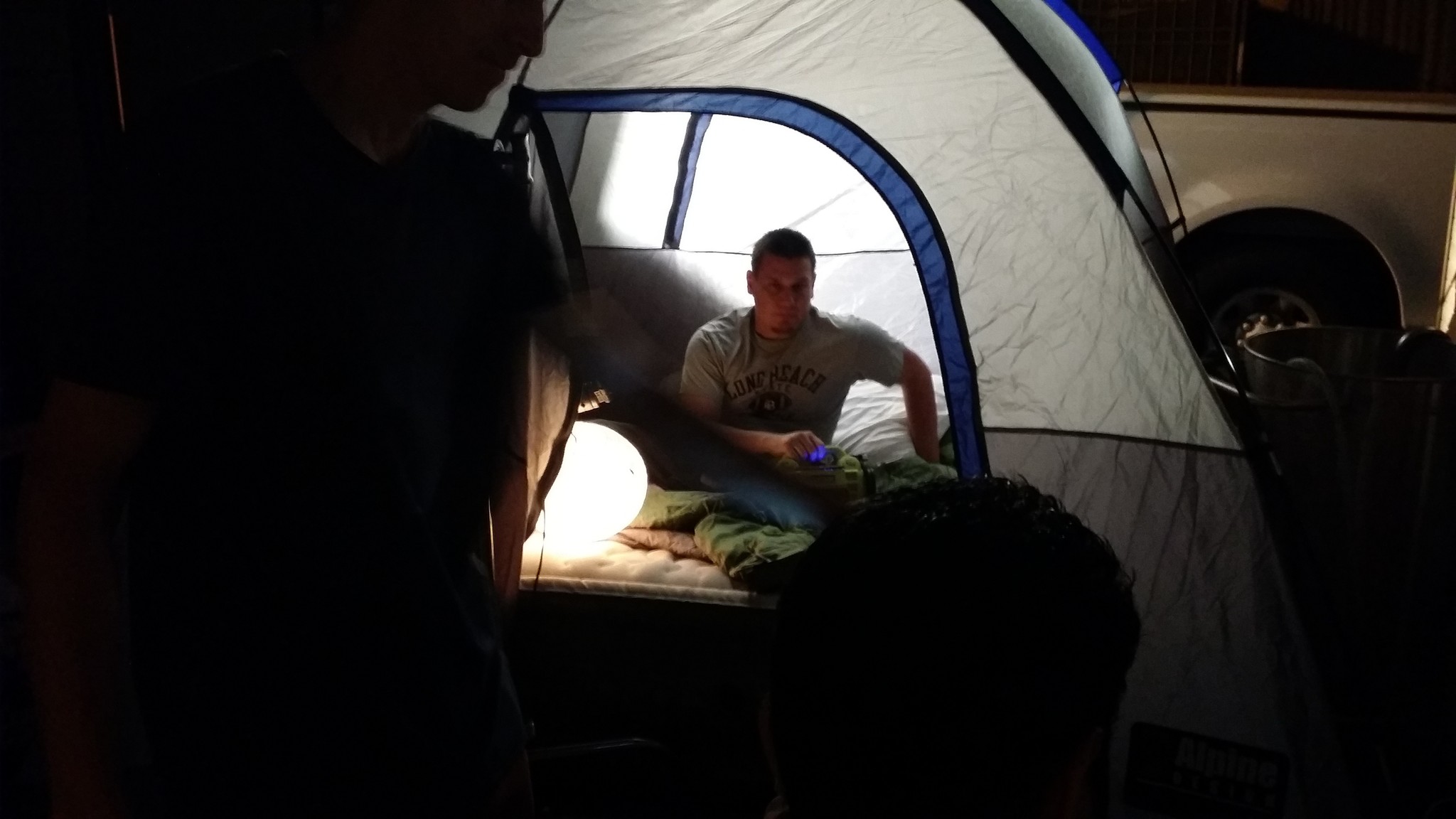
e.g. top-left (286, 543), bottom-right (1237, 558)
top-left (1114, 83), bottom-right (1456, 417)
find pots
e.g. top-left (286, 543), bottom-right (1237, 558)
top-left (1240, 320), bottom-right (1455, 574)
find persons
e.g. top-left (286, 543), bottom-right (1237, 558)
top-left (5, 2), bottom-right (718, 817)
top-left (708, 478), bottom-right (1147, 817)
top-left (676, 228), bottom-right (944, 478)
